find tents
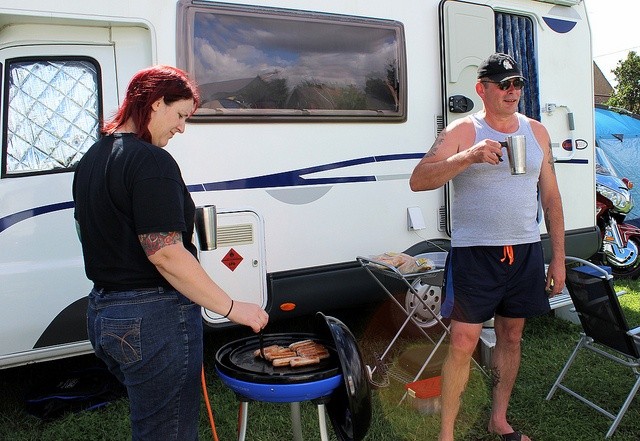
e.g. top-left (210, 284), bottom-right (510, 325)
top-left (595, 105), bottom-right (640, 223)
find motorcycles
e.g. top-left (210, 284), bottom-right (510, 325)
top-left (596, 146), bottom-right (640, 278)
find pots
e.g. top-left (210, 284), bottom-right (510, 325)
top-left (214, 332), bottom-right (338, 404)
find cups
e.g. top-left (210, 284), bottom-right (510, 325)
top-left (494, 136), bottom-right (527, 175)
top-left (195, 205), bottom-right (218, 251)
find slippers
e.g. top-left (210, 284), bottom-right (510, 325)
top-left (499, 430), bottom-right (531, 441)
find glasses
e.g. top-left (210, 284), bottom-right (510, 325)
top-left (478, 79), bottom-right (524, 91)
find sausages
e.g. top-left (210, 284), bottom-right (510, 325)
top-left (289, 338), bottom-right (330, 359)
top-left (254, 344), bottom-right (279, 357)
top-left (272, 356), bottom-right (301, 367)
top-left (290, 356), bottom-right (320, 368)
top-left (265, 347), bottom-right (297, 362)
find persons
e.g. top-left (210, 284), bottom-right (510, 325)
top-left (410, 52), bottom-right (567, 441)
top-left (72, 64), bottom-right (272, 441)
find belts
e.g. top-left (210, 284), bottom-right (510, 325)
top-left (92, 283), bottom-right (176, 293)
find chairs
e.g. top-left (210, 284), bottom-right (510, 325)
top-left (546, 256), bottom-right (640, 439)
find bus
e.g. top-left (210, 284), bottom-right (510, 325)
top-left (2, 0), bottom-right (599, 376)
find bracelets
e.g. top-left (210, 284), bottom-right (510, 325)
top-left (223, 300), bottom-right (234, 319)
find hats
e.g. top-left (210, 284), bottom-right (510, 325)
top-left (476, 53), bottom-right (529, 84)
